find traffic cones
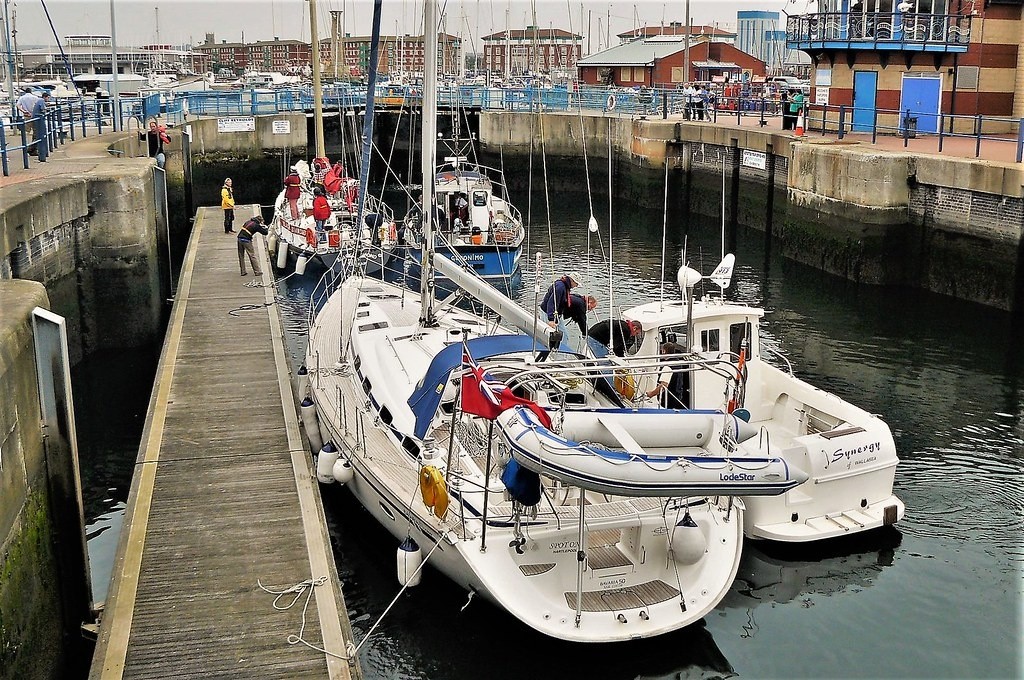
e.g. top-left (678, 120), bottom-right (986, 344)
top-left (793, 110), bottom-right (809, 138)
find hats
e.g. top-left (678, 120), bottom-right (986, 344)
top-left (566, 272), bottom-right (582, 286)
top-left (290, 166), bottom-right (297, 172)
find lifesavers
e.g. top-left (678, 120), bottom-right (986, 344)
top-left (388, 222), bottom-right (397, 241)
top-left (614, 366), bottom-right (634, 403)
top-left (606, 94), bottom-right (617, 111)
top-left (306, 227), bottom-right (315, 245)
top-left (420, 464), bottom-right (451, 520)
top-left (182, 99), bottom-right (189, 115)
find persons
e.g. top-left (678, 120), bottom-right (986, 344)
top-left (782, 89), bottom-right (804, 131)
top-left (454, 193), bottom-right (468, 220)
top-left (237, 215), bottom-right (268, 275)
top-left (312, 188), bottom-right (331, 241)
top-left (221, 177), bottom-right (236, 234)
top-left (365, 213), bottom-right (382, 240)
top-left (16, 88), bottom-right (39, 144)
top-left (562, 293), bottom-right (597, 339)
top-left (853, 0), bottom-right (863, 37)
top-left (540, 271), bottom-right (583, 346)
top-left (405, 195), bottom-right (422, 221)
top-left (311, 158), bottom-right (330, 184)
top-left (28, 92), bottom-right (50, 149)
top-left (587, 319), bottom-right (642, 388)
top-left (646, 343), bottom-right (683, 409)
top-left (284, 166), bottom-right (301, 221)
top-left (725, 84), bottom-right (741, 97)
top-left (683, 83), bottom-right (711, 122)
top-left (137, 122), bottom-right (171, 168)
top-left (333, 160), bottom-right (342, 176)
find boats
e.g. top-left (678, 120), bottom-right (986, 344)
top-left (620, 156), bottom-right (906, 543)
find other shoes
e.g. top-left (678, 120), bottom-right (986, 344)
top-left (255, 272), bottom-right (263, 276)
top-left (241, 273), bottom-right (247, 276)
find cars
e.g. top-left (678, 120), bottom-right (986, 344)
top-left (771, 76), bottom-right (810, 95)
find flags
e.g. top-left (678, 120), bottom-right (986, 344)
top-left (461, 342), bottom-right (554, 430)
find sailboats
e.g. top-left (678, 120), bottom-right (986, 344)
top-left (300, 0), bottom-right (810, 643)
top-left (391, 0), bottom-right (526, 277)
top-left (272, 0), bottom-right (400, 276)
top-left (0, 0), bottom-right (178, 132)
